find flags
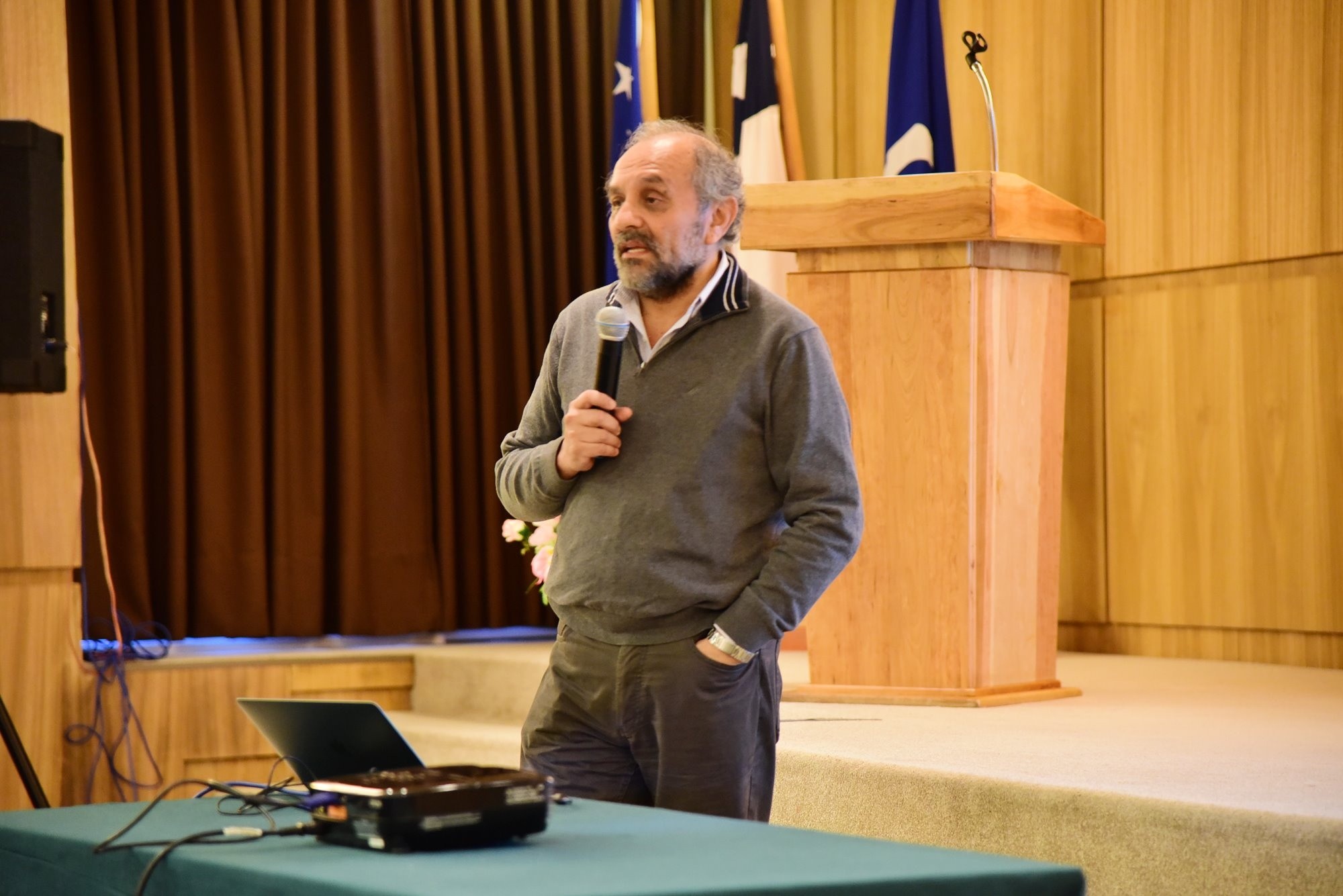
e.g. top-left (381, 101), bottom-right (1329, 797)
top-left (732, 0), bottom-right (789, 185)
top-left (883, 1), bottom-right (956, 176)
top-left (608, 0), bottom-right (642, 283)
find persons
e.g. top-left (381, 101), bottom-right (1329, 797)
top-left (493, 121), bottom-right (864, 824)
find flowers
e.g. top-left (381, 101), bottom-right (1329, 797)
top-left (502, 515), bottom-right (564, 604)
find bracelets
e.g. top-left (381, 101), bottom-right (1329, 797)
top-left (706, 628), bottom-right (752, 665)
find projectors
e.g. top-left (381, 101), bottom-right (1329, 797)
top-left (309, 764), bottom-right (549, 852)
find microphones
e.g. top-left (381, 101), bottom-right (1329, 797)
top-left (591, 306), bottom-right (630, 461)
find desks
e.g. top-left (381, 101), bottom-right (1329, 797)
top-left (0, 794), bottom-right (1088, 896)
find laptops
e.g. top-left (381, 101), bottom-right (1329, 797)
top-left (239, 698), bottom-right (427, 784)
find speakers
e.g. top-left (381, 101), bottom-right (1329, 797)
top-left (0, 118), bottom-right (68, 395)
